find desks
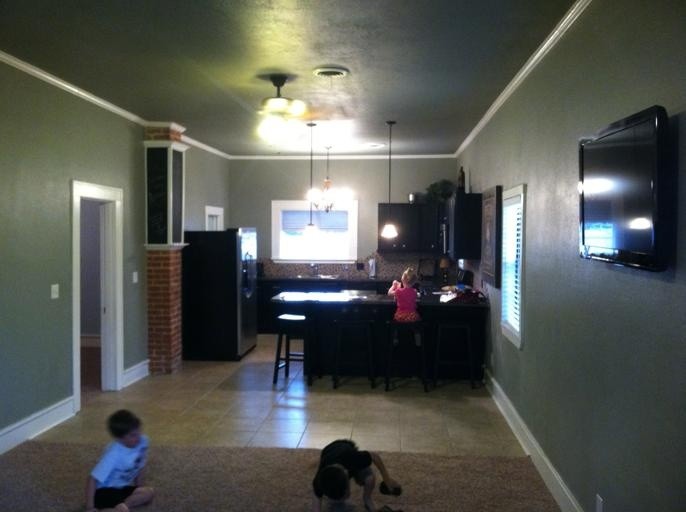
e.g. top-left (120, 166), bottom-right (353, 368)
top-left (269, 284), bottom-right (488, 384)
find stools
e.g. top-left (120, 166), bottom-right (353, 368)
top-left (272, 311), bottom-right (475, 396)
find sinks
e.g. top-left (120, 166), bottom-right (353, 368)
top-left (296, 274), bottom-right (339, 279)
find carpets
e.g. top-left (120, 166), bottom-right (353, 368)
top-left (0, 440), bottom-right (565, 512)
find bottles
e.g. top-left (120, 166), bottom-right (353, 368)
top-left (368, 255), bottom-right (376, 280)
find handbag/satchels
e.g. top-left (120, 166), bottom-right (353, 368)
top-left (453, 287), bottom-right (487, 304)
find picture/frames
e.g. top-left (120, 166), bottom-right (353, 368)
top-left (480, 182), bottom-right (503, 289)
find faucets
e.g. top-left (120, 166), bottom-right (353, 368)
top-left (311, 264), bottom-right (318, 275)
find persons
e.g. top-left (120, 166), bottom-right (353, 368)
top-left (386, 266), bottom-right (424, 348)
top-left (309, 437), bottom-right (404, 512)
top-left (83, 408), bottom-right (157, 512)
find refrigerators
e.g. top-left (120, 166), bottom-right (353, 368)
top-left (181, 226), bottom-right (257, 361)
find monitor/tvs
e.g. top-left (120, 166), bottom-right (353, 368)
top-left (578, 105), bottom-right (671, 272)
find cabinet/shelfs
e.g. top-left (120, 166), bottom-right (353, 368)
top-left (378, 192), bottom-right (482, 261)
